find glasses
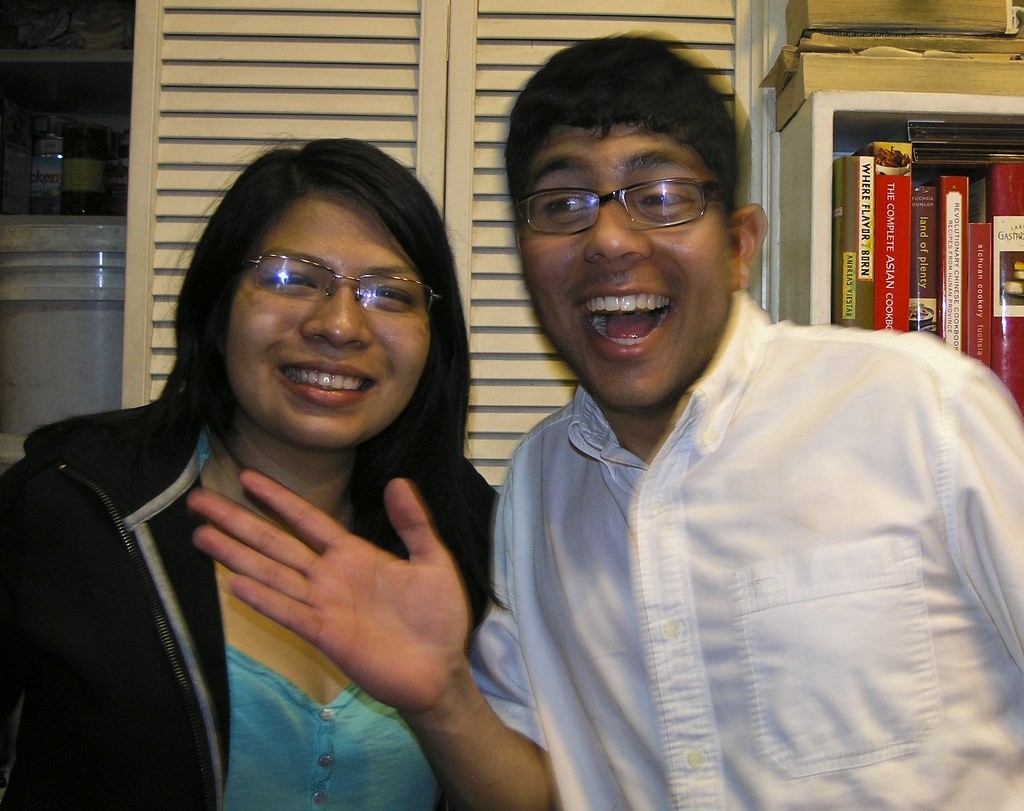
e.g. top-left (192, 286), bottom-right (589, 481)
top-left (240, 255), bottom-right (442, 317)
top-left (518, 177), bottom-right (731, 236)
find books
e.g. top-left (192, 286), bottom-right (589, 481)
top-left (757, 0), bottom-right (1024, 418)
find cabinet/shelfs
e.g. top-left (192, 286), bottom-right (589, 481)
top-left (770, 91), bottom-right (1024, 325)
top-left (0, 48), bottom-right (134, 227)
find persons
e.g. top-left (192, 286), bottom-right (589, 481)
top-left (0, 139), bottom-right (499, 811)
top-left (191, 37), bottom-right (1024, 811)
